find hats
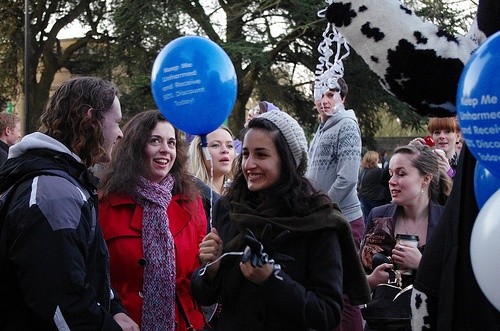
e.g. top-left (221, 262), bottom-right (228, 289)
top-left (253, 110), bottom-right (309, 176)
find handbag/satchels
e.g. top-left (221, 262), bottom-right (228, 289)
top-left (361, 252), bottom-right (416, 321)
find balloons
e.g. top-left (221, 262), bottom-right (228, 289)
top-left (151, 36), bottom-right (238, 148)
top-left (456, 30), bottom-right (500, 312)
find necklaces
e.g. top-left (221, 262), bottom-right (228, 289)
top-left (404, 214), bottom-right (428, 236)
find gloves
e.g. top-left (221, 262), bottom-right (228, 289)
top-left (260, 223), bottom-right (295, 280)
top-left (240, 227), bottom-right (266, 267)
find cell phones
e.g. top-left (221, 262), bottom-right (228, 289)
top-left (421, 136), bottom-right (435, 147)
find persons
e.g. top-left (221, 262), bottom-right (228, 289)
top-left (97, 109), bottom-right (208, 331)
top-left (185, 101), bottom-right (281, 155)
top-left (0, 77), bottom-right (141, 331)
top-left (303, 76), bottom-right (365, 331)
top-left (191, 110), bottom-right (371, 331)
top-left (360, 142), bottom-right (445, 331)
top-left (184, 125), bottom-right (241, 235)
top-left (354, 115), bottom-right (465, 224)
top-left (0, 111), bottom-right (21, 167)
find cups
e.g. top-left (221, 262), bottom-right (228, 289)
top-left (431, 149), bottom-right (446, 160)
top-left (396, 233), bottom-right (419, 276)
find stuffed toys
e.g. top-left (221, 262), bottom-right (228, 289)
top-left (325, 0), bottom-right (500, 331)
